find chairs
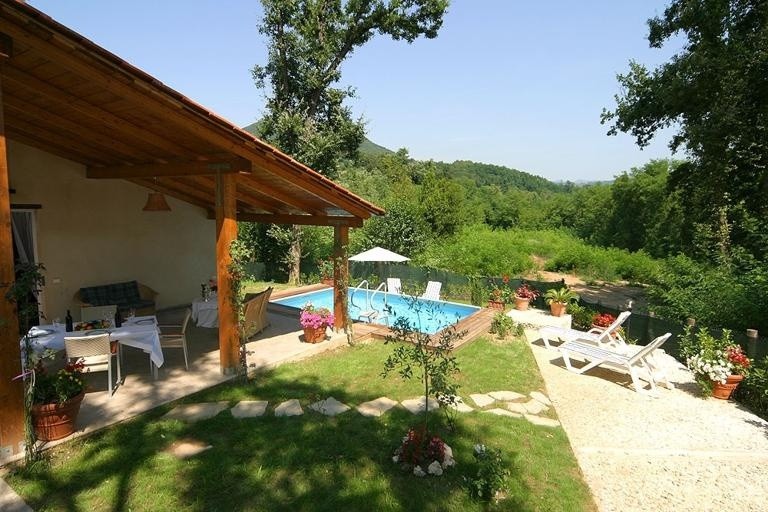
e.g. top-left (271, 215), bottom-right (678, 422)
top-left (422, 281), bottom-right (442, 300)
top-left (387, 278), bottom-right (401, 293)
top-left (539, 311), bottom-right (672, 394)
top-left (64, 333), bottom-right (121, 397)
top-left (143, 308), bottom-right (191, 377)
top-left (82, 304), bottom-right (116, 326)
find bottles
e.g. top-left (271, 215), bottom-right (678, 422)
top-left (66, 309), bottom-right (73, 332)
top-left (115, 304), bottom-right (121, 327)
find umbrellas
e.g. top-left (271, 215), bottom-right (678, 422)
top-left (347, 245), bottom-right (413, 280)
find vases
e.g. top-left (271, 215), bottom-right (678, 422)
top-left (304, 327), bottom-right (326, 343)
top-left (712, 374), bottom-right (743, 400)
top-left (489, 300), bottom-right (505, 311)
top-left (30, 390), bottom-right (85, 441)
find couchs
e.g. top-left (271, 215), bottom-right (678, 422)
top-left (73, 279), bottom-right (159, 327)
top-left (241, 287), bottom-right (273, 340)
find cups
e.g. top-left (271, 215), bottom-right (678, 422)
top-left (53, 319), bottom-right (59, 331)
top-left (128, 309), bottom-right (136, 325)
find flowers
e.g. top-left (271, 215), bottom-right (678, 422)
top-left (686, 343), bottom-right (751, 384)
top-left (489, 274), bottom-right (510, 302)
top-left (299, 301), bottom-right (335, 329)
top-left (28, 346), bottom-right (90, 404)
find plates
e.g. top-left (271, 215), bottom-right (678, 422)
top-left (85, 329), bottom-right (109, 337)
top-left (135, 318), bottom-right (153, 325)
top-left (29, 328), bottom-right (53, 338)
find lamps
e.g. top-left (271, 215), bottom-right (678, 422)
top-left (143, 176), bottom-right (171, 211)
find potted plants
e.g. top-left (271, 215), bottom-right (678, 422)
top-left (513, 283), bottom-right (580, 316)
